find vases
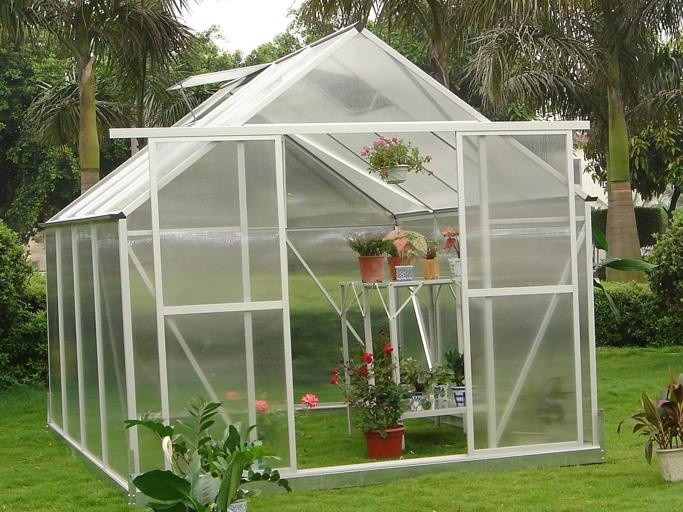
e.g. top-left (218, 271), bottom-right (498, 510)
top-left (380, 165), bottom-right (408, 184)
top-left (364, 426), bottom-right (405, 457)
top-left (447, 256), bottom-right (460, 276)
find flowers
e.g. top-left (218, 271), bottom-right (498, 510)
top-left (359, 136), bottom-right (434, 183)
top-left (329, 329), bottom-right (412, 441)
top-left (219, 385), bottom-right (318, 434)
top-left (440, 226), bottom-right (461, 258)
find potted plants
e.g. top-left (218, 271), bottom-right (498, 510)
top-left (342, 227), bottom-right (443, 282)
top-left (615, 359), bottom-right (683, 484)
top-left (124, 397), bottom-right (294, 512)
top-left (400, 346), bottom-right (468, 411)
